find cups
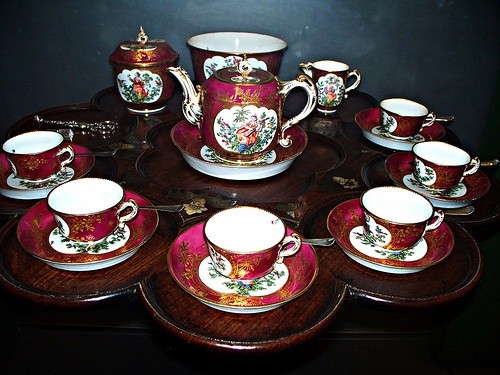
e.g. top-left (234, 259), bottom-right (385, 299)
top-left (359, 186), bottom-right (444, 253)
top-left (380, 98), bottom-right (436, 140)
top-left (98, 224), bottom-right (101, 225)
top-left (412, 141), bottom-right (480, 192)
top-left (110, 26), bottom-right (180, 113)
top-left (2, 131), bottom-right (74, 182)
top-left (299, 60), bottom-right (361, 114)
top-left (46, 177), bottom-right (139, 242)
top-left (186, 32), bottom-right (288, 91)
top-left (203, 206), bottom-right (302, 280)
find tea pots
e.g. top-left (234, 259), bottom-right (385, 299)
top-left (165, 53), bottom-right (317, 163)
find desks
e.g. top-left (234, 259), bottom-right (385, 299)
top-left (0, 87), bottom-right (500, 375)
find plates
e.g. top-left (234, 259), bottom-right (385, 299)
top-left (17, 189), bottom-right (159, 271)
top-left (0, 142), bottom-right (96, 200)
top-left (170, 117), bottom-right (309, 181)
top-left (354, 108), bottom-right (446, 151)
top-left (384, 151), bottom-right (490, 208)
top-left (167, 220), bottom-right (319, 314)
top-left (326, 198), bottom-right (454, 274)
